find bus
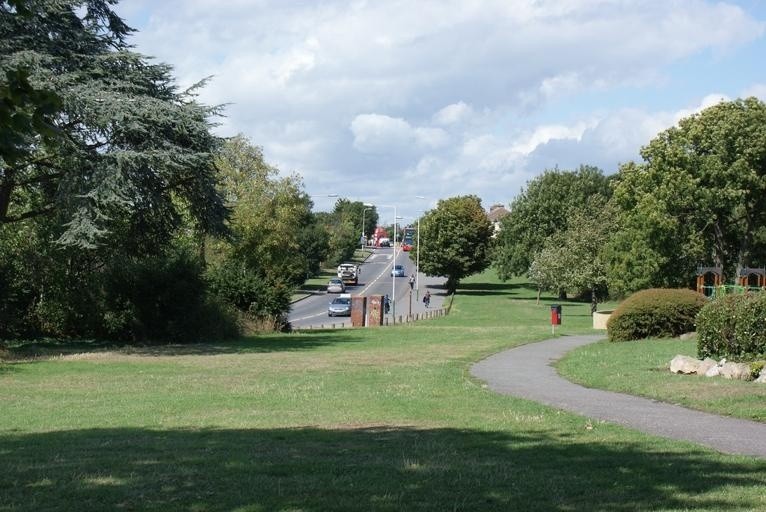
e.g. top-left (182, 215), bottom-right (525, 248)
top-left (402, 227), bottom-right (415, 251)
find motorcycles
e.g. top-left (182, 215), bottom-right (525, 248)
top-left (383, 298), bottom-right (391, 314)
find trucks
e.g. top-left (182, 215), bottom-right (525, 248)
top-left (337, 261), bottom-right (361, 285)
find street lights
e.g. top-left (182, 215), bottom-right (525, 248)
top-left (361, 208), bottom-right (373, 257)
top-left (363, 203), bottom-right (397, 320)
top-left (416, 196), bottom-right (436, 210)
top-left (395, 215), bottom-right (418, 301)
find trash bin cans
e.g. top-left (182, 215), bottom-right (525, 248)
top-left (551, 305), bottom-right (561, 325)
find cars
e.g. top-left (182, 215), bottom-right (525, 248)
top-left (391, 264), bottom-right (405, 277)
top-left (328, 296), bottom-right (351, 316)
top-left (379, 237), bottom-right (390, 247)
top-left (327, 278), bottom-right (346, 293)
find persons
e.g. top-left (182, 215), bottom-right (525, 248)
top-left (384, 293), bottom-right (392, 314)
top-left (407, 273), bottom-right (417, 291)
top-left (423, 290), bottom-right (432, 309)
